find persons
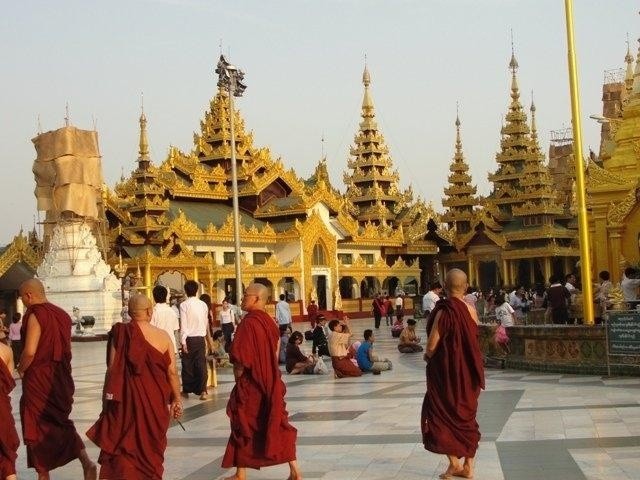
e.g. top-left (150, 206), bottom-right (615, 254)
top-left (422, 283), bottom-right (442, 317)
top-left (85, 294), bottom-right (184, 479)
top-left (152, 280), bottom-right (243, 400)
top-left (419, 268), bottom-right (486, 480)
top-left (0, 310), bottom-right (10, 347)
top-left (273, 293), bottom-right (394, 378)
top-left (463, 274), bottom-right (576, 328)
top-left (597, 268), bottom-right (640, 300)
top-left (373, 294), bottom-right (423, 354)
top-left (220, 284), bottom-right (301, 480)
top-left (16, 278), bottom-right (97, 480)
top-left (9, 313), bottom-right (23, 370)
top-left (0, 341), bottom-right (21, 479)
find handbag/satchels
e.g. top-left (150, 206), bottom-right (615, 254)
top-left (303, 355), bottom-right (315, 374)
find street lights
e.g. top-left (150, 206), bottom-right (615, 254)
top-left (215, 54), bottom-right (247, 305)
